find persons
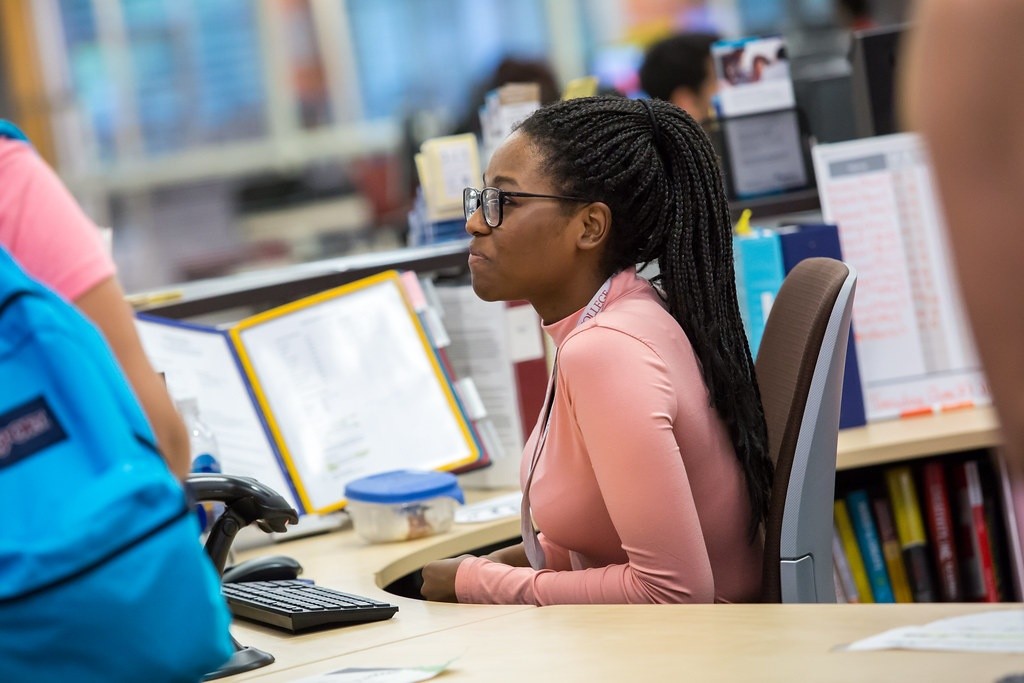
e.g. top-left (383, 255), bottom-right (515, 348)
top-left (420, 94), bottom-right (775, 602)
top-left (2, 124), bottom-right (194, 488)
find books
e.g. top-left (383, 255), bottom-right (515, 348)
top-left (130, 257), bottom-right (560, 518)
top-left (835, 454), bottom-right (1016, 602)
top-left (733, 130), bottom-right (995, 432)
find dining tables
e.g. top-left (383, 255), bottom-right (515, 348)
top-left (211, 406), bottom-right (1023, 683)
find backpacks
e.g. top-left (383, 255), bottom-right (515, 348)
top-left (2, 247), bottom-right (230, 683)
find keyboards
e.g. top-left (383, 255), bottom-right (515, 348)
top-left (221, 579), bottom-right (399, 634)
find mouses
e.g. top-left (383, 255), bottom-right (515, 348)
top-left (221, 555), bottom-right (302, 582)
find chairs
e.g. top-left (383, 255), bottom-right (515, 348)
top-left (748, 255), bottom-right (860, 604)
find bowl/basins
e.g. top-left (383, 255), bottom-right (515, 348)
top-left (343, 468), bottom-right (465, 544)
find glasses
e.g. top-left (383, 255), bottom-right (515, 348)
top-left (463, 187), bottom-right (594, 227)
